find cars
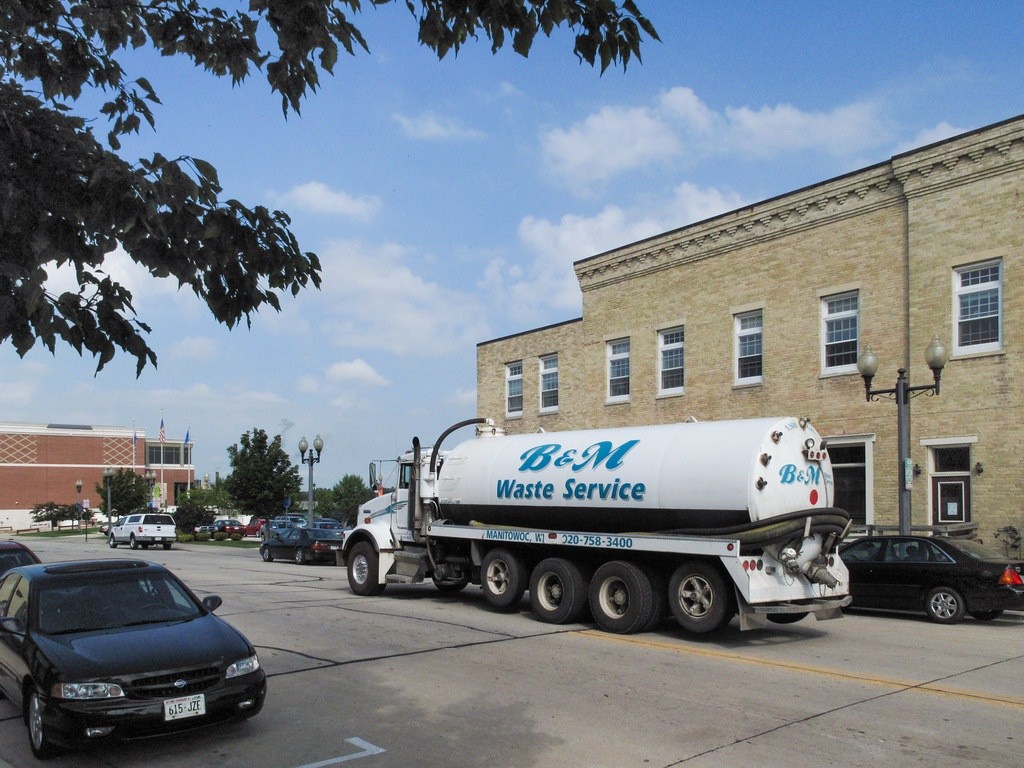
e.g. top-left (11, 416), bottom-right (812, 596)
top-left (259, 527), bottom-right (343, 564)
top-left (244, 519), bottom-right (265, 537)
top-left (259, 520), bottom-right (299, 541)
top-left (275, 516), bottom-right (308, 528)
top-left (194, 524), bottom-right (218, 533)
top-left (99, 518), bottom-right (123, 536)
top-left (839, 535), bottom-right (1024, 625)
top-left (0, 538), bottom-right (43, 577)
top-left (0, 558), bottom-right (267, 760)
top-left (214, 520), bottom-right (247, 538)
top-left (302, 518), bottom-right (341, 529)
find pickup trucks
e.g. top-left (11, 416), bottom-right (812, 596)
top-left (109, 513), bottom-right (177, 550)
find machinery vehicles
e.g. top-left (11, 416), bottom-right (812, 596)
top-left (340, 417), bottom-right (854, 638)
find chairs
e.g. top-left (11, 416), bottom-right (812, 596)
top-left (902, 545), bottom-right (918, 562)
top-left (881, 542), bottom-right (899, 562)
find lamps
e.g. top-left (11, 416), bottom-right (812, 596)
top-left (975, 462), bottom-right (983, 473)
top-left (914, 464), bottom-right (920, 475)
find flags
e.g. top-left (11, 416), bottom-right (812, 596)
top-left (184, 429), bottom-right (189, 445)
top-left (134, 430), bottom-right (137, 447)
top-left (159, 419), bottom-right (165, 444)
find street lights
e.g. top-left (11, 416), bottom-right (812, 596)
top-left (76, 479), bottom-right (83, 530)
top-left (298, 434), bottom-right (323, 529)
top-left (104, 466), bottom-right (113, 544)
top-left (857, 334), bottom-right (949, 559)
top-left (145, 470), bottom-right (157, 513)
